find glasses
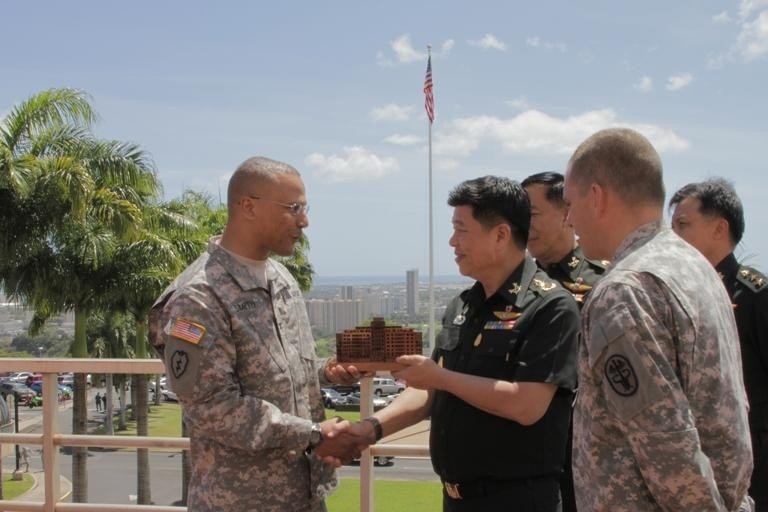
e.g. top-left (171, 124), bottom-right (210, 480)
top-left (237, 195), bottom-right (310, 216)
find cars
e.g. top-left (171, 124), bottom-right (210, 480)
top-left (0, 373), bottom-right (93, 402)
top-left (150, 378), bottom-right (178, 402)
top-left (319, 379), bottom-right (406, 408)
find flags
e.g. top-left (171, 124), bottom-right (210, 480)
top-left (421, 55), bottom-right (435, 124)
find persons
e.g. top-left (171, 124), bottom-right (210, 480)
top-left (562, 126), bottom-right (757, 512)
top-left (95, 392), bottom-right (102, 412)
top-left (146, 155), bottom-right (374, 511)
top-left (312, 175), bottom-right (581, 512)
top-left (521, 172), bottom-right (608, 511)
top-left (667, 177), bottom-right (768, 512)
top-left (19, 446), bottom-right (35, 473)
top-left (102, 393), bottom-right (106, 412)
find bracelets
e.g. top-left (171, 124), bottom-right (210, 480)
top-left (362, 416), bottom-right (383, 441)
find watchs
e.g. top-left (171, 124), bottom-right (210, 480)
top-left (305, 422), bottom-right (325, 457)
